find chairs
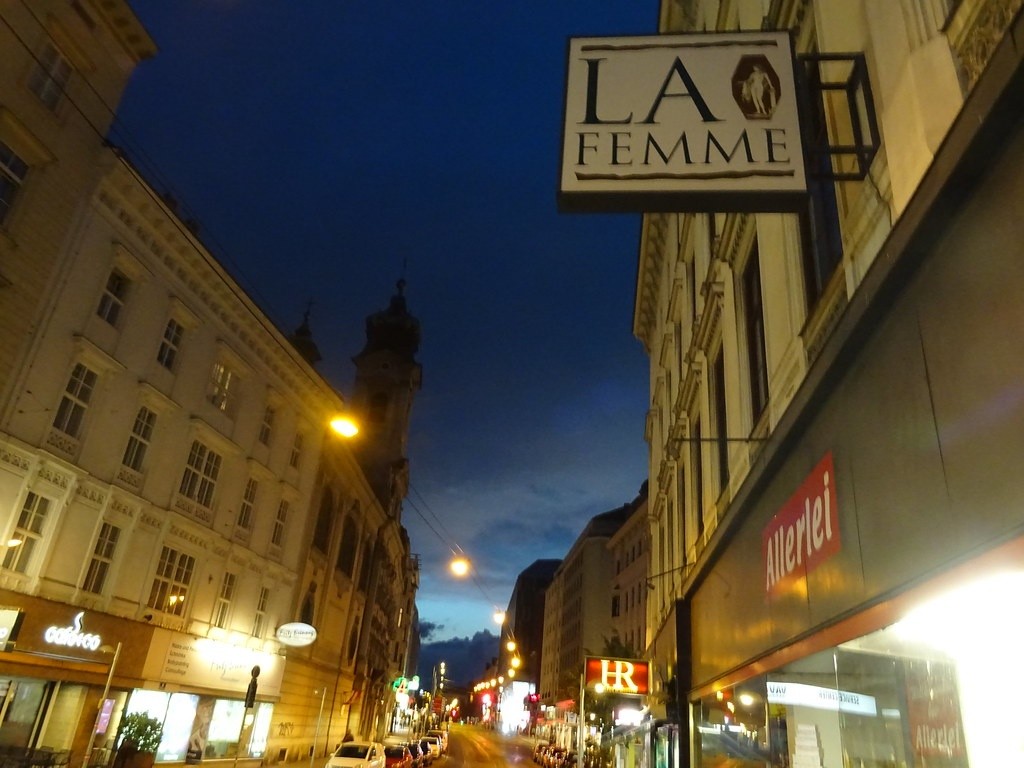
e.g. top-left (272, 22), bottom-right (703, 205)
top-left (0, 745), bottom-right (74, 768)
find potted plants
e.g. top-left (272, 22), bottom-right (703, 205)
top-left (111, 709), bottom-right (166, 768)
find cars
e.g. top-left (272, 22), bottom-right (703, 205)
top-left (402, 730), bottom-right (448, 768)
top-left (324, 741), bottom-right (386, 768)
top-left (384, 745), bottom-right (413, 768)
top-left (532, 740), bottom-right (581, 768)
